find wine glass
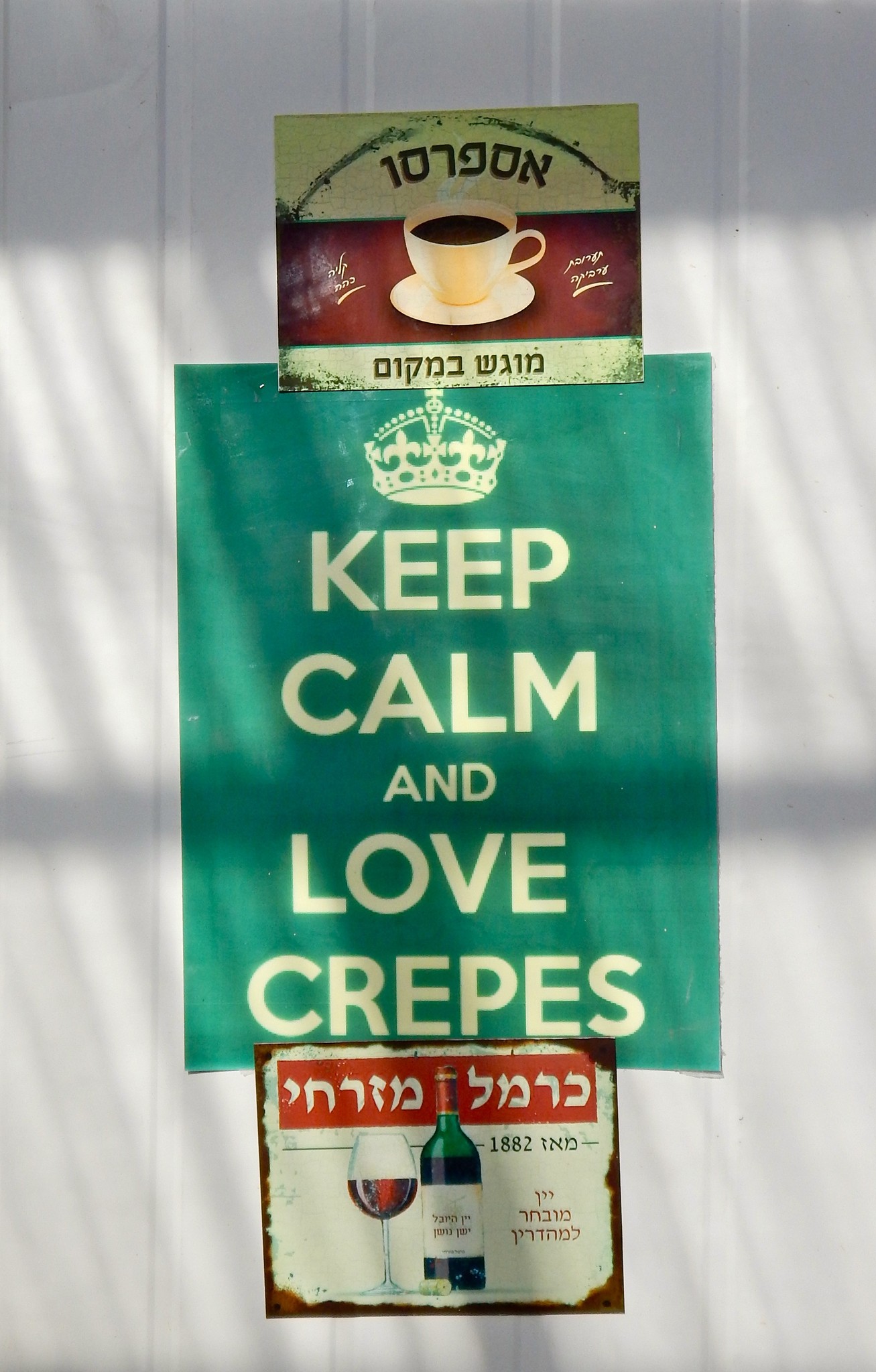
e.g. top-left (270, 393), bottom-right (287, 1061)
top-left (347, 1132), bottom-right (418, 1297)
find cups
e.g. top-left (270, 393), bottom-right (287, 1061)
top-left (402, 199), bottom-right (547, 307)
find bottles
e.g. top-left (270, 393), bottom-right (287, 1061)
top-left (420, 1066), bottom-right (487, 1291)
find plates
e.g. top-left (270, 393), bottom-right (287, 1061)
top-left (390, 272), bottom-right (535, 325)
